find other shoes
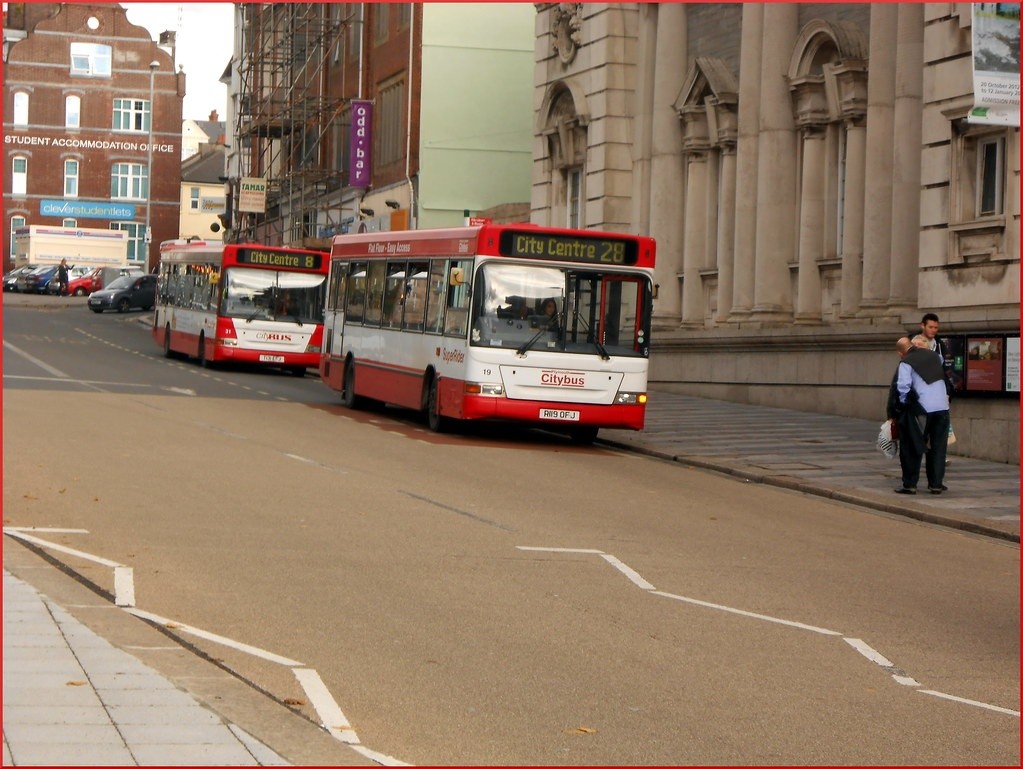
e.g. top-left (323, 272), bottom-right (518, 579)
top-left (928, 482), bottom-right (948, 491)
top-left (930, 487), bottom-right (942, 495)
top-left (895, 486), bottom-right (917, 495)
top-left (945, 460), bottom-right (953, 467)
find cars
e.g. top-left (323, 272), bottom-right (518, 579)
top-left (2, 264), bottom-right (145, 296)
top-left (87, 274), bottom-right (158, 314)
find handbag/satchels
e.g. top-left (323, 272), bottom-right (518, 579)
top-left (877, 419), bottom-right (900, 460)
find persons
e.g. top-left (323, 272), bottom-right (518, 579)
top-left (529, 298), bottom-right (559, 332)
top-left (887, 313), bottom-right (953, 495)
top-left (58, 258), bottom-right (74, 297)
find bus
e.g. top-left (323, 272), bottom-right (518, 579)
top-left (152, 236), bottom-right (332, 377)
top-left (318, 223), bottom-right (660, 448)
